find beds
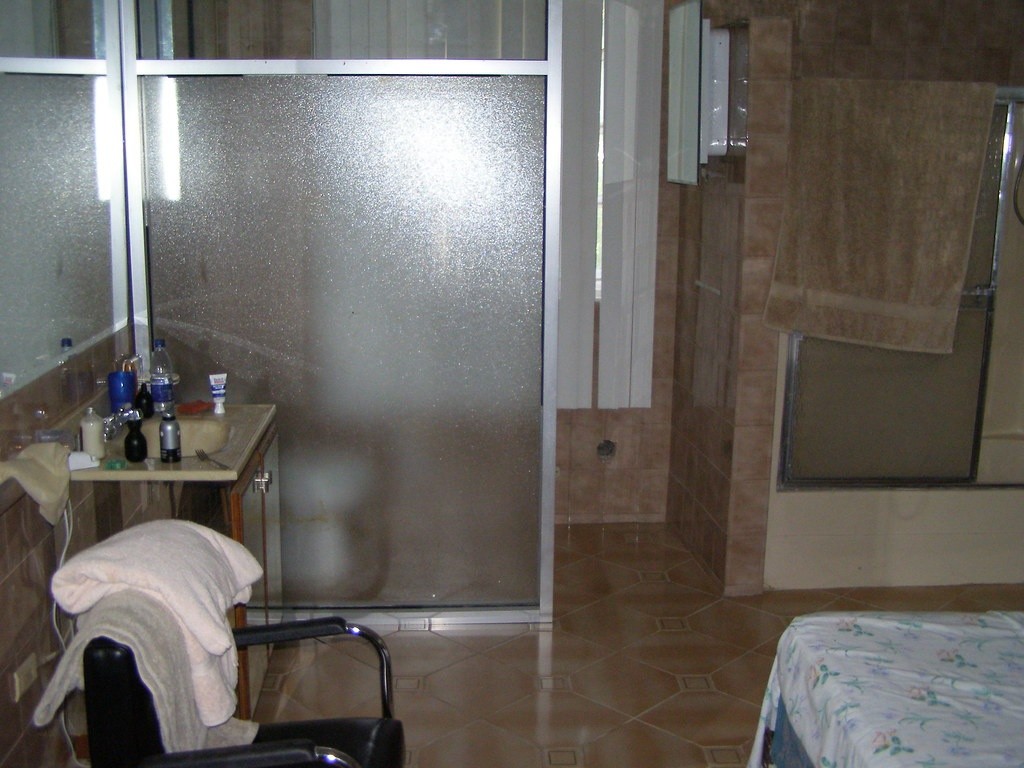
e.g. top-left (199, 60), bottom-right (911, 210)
top-left (747, 609), bottom-right (1024, 768)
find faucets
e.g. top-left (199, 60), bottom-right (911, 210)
top-left (104, 408), bottom-right (143, 443)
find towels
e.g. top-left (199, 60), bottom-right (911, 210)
top-left (763, 78), bottom-right (997, 354)
top-left (33, 519), bottom-right (263, 754)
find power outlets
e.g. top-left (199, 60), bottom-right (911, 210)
top-left (12, 652), bottom-right (38, 702)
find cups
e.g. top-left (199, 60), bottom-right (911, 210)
top-left (108, 370), bottom-right (136, 414)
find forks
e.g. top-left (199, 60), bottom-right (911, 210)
top-left (195, 449), bottom-right (230, 470)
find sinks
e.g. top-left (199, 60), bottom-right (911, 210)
top-left (116, 417), bottom-right (231, 458)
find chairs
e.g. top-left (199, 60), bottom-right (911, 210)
top-left (82, 614), bottom-right (404, 768)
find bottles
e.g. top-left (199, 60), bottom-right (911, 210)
top-left (149, 338), bottom-right (175, 416)
top-left (159, 411), bottom-right (181, 463)
top-left (78, 407), bottom-right (107, 459)
top-left (124, 419), bottom-right (148, 463)
top-left (134, 382), bottom-right (154, 418)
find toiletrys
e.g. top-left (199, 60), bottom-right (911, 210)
top-left (209, 373), bottom-right (227, 413)
